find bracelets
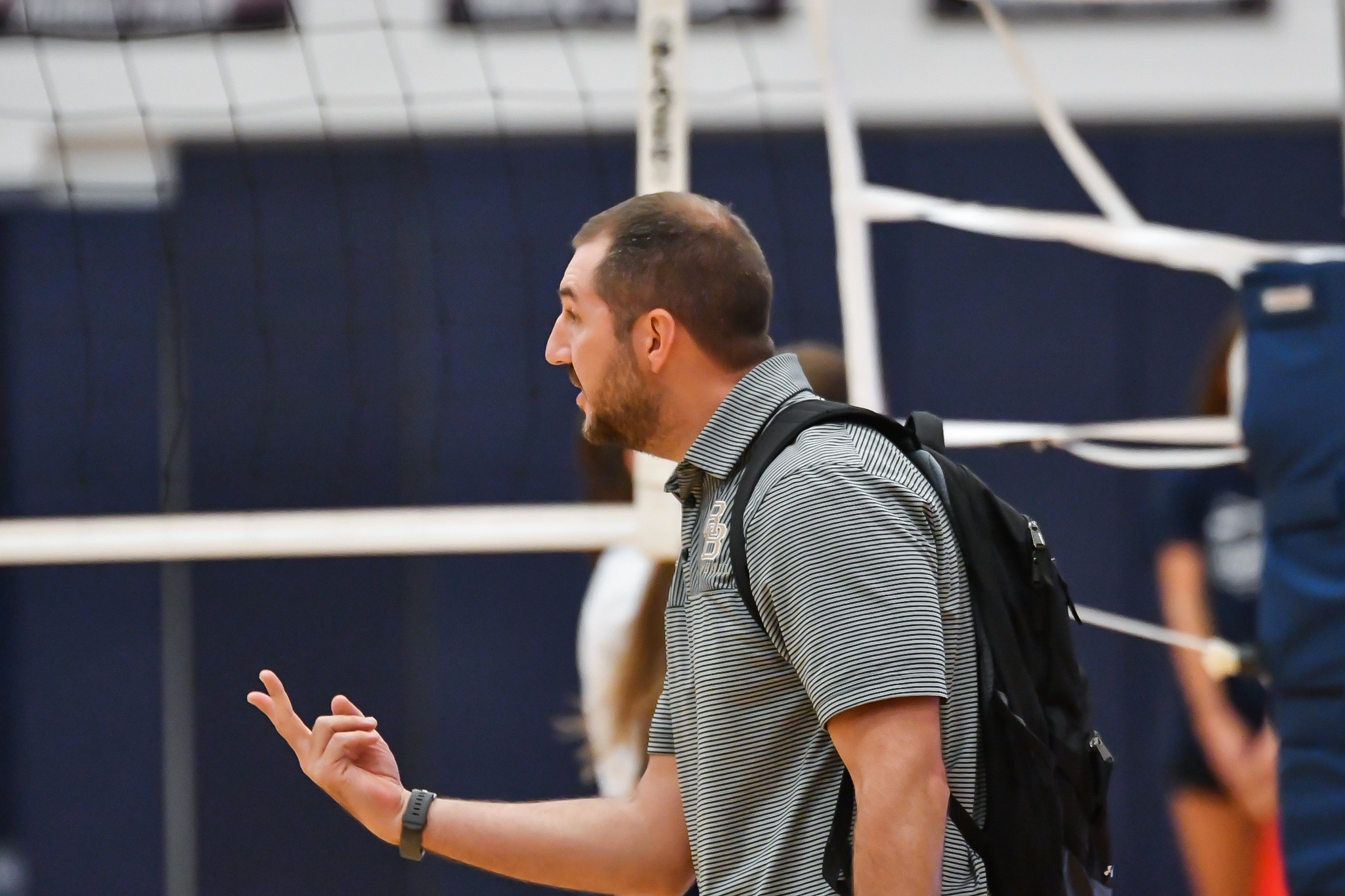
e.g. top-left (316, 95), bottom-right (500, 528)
top-left (399, 788), bottom-right (437, 862)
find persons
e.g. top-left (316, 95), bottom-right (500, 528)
top-left (242, 193), bottom-right (994, 896)
top-left (1156, 303), bottom-right (1292, 896)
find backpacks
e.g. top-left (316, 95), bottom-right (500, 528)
top-left (728, 400), bottom-right (1111, 896)
